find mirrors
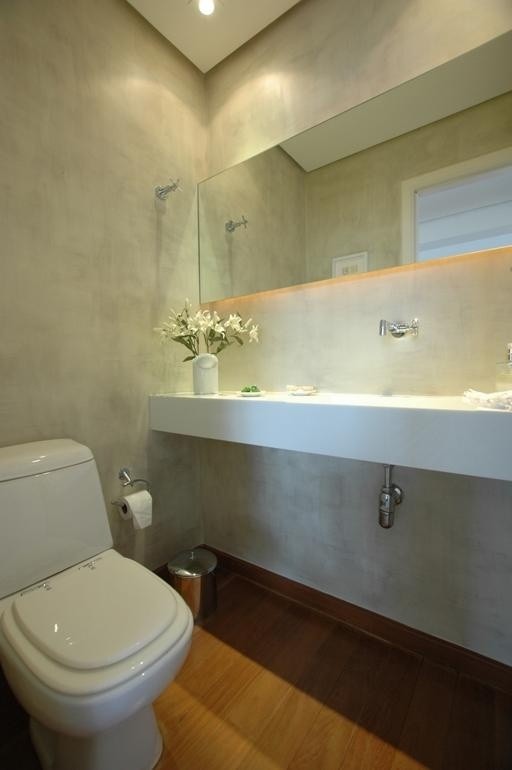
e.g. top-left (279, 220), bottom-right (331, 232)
top-left (197, 31), bottom-right (512, 302)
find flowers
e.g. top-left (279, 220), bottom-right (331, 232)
top-left (153, 298), bottom-right (259, 362)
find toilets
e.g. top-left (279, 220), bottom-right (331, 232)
top-left (0, 438), bottom-right (195, 770)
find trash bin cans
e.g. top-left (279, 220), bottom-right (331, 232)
top-left (167, 550), bottom-right (218, 621)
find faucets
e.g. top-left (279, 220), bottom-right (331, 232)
top-left (378, 317), bottom-right (419, 338)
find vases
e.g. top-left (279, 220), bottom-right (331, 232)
top-left (192, 353), bottom-right (219, 394)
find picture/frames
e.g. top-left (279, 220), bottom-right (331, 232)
top-left (331, 251), bottom-right (368, 278)
